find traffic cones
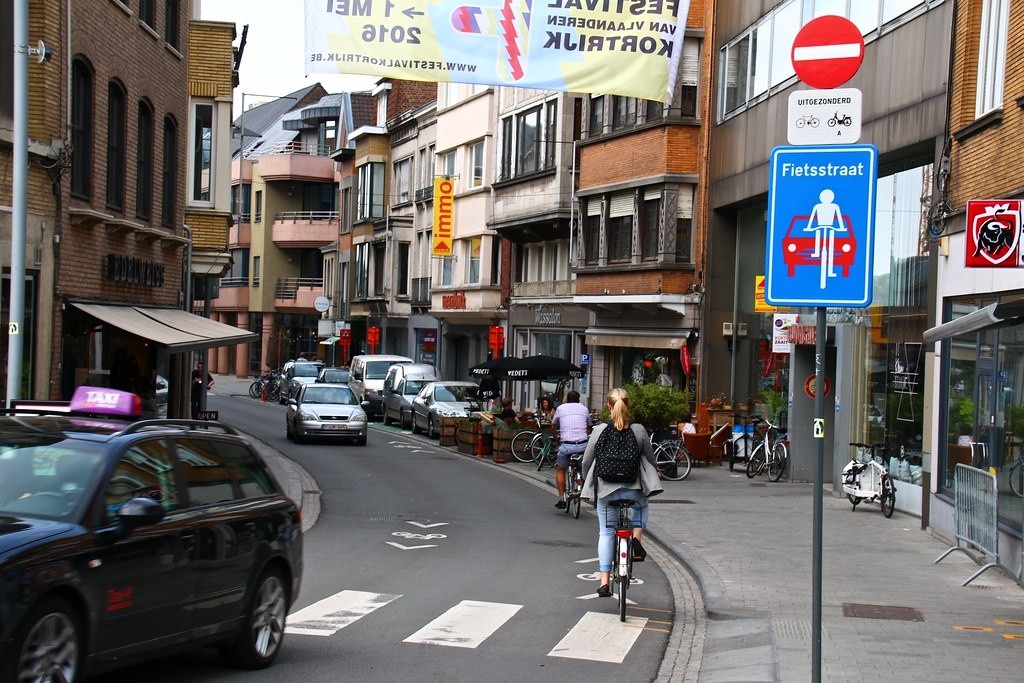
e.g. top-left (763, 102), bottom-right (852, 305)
top-left (261, 390), bottom-right (268, 404)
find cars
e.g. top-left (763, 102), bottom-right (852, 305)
top-left (410, 380), bottom-right (479, 441)
top-left (315, 367), bottom-right (350, 386)
top-left (286, 382), bottom-right (368, 448)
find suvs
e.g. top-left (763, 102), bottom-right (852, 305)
top-left (278, 358), bottom-right (326, 405)
top-left (0, 385), bottom-right (304, 683)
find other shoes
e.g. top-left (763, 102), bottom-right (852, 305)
top-left (632, 537), bottom-right (647, 561)
top-left (597, 584), bottom-right (609, 593)
top-left (555, 501), bottom-right (566, 510)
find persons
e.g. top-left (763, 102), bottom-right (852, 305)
top-left (501, 397), bottom-right (521, 424)
top-left (550, 390), bottom-right (593, 507)
top-left (582, 387), bottom-right (664, 595)
top-left (191, 362), bottom-right (214, 413)
top-left (539, 398), bottom-right (555, 418)
top-left (297, 352), bottom-right (322, 363)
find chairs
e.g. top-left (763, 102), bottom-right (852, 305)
top-left (57, 455), bottom-right (85, 484)
top-left (709, 422), bottom-right (730, 466)
top-left (683, 431), bottom-right (712, 467)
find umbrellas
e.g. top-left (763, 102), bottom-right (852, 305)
top-left (468, 353), bottom-right (584, 410)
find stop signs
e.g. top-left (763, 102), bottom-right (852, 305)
top-left (789, 16), bottom-right (863, 88)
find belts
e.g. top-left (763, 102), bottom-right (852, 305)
top-left (563, 440), bottom-right (588, 444)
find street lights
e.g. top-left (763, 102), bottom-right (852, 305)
top-left (239, 92), bottom-right (297, 221)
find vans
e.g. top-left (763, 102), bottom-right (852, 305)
top-left (348, 354), bottom-right (414, 421)
top-left (381, 362), bottom-right (444, 430)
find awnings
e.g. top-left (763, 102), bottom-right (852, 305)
top-left (68, 299), bottom-right (259, 353)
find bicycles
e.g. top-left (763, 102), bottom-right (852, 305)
top-left (745, 417), bottom-right (788, 482)
top-left (510, 415), bottom-right (693, 520)
top-left (604, 498), bottom-right (641, 622)
top-left (843, 441), bottom-right (897, 519)
top-left (249, 364), bottom-right (280, 403)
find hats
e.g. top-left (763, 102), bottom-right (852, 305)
top-left (501, 397), bottom-right (514, 408)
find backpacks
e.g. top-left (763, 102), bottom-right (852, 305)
top-left (594, 420), bottom-right (640, 485)
